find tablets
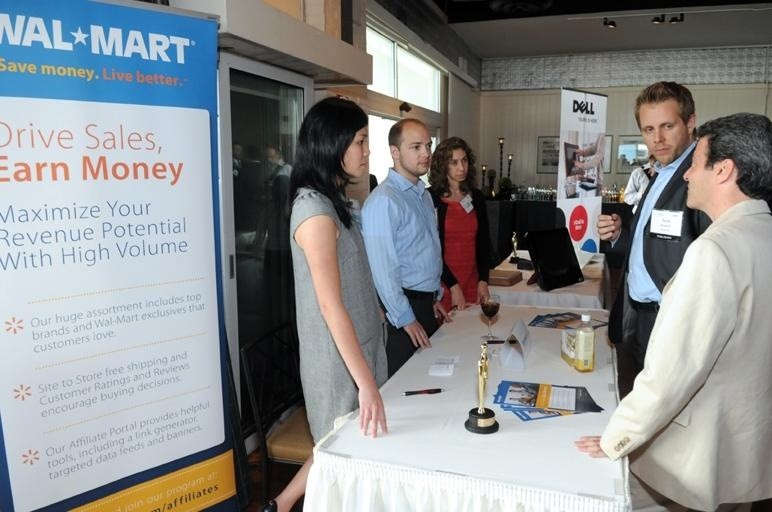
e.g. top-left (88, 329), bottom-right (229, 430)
top-left (524, 228), bottom-right (584, 290)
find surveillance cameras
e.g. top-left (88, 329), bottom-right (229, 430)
top-left (399, 102), bottom-right (411, 114)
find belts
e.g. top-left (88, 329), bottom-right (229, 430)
top-left (628, 294), bottom-right (660, 313)
top-left (405, 291), bottom-right (437, 300)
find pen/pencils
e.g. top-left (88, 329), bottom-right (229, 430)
top-left (401, 388), bottom-right (445, 396)
top-left (481, 340), bottom-right (517, 344)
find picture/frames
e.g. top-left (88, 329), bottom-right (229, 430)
top-left (536, 133), bottom-right (651, 176)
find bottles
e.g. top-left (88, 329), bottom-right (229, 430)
top-left (620, 187), bottom-right (625, 203)
top-left (575, 312), bottom-right (597, 374)
top-left (528, 185), bottom-right (618, 202)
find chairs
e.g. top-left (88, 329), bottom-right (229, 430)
top-left (234, 319), bottom-right (316, 510)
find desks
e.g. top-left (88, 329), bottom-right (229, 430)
top-left (480, 246), bottom-right (610, 310)
top-left (298, 305), bottom-right (635, 512)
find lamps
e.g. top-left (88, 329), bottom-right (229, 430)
top-left (477, 129), bottom-right (516, 190)
top-left (569, 6), bottom-right (772, 29)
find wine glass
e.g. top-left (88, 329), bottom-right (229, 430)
top-left (479, 292), bottom-right (505, 343)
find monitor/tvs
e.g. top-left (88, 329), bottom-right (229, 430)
top-left (563, 141), bottom-right (585, 177)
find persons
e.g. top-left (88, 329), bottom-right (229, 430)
top-left (596, 81), bottom-right (713, 403)
top-left (574, 118), bottom-right (606, 198)
top-left (429, 136), bottom-right (490, 311)
top-left (361, 119), bottom-right (451, 377)
top-left (508, 382), bottom-right (538, 406)
top-left (623, 154), bottom-right (657, 212)
top-left (265, 97), bottom-right (388, 510)
top-left (232, 142), bottom-right (293, 333)
top-left (573, 111), bottom-right (771, 511)
